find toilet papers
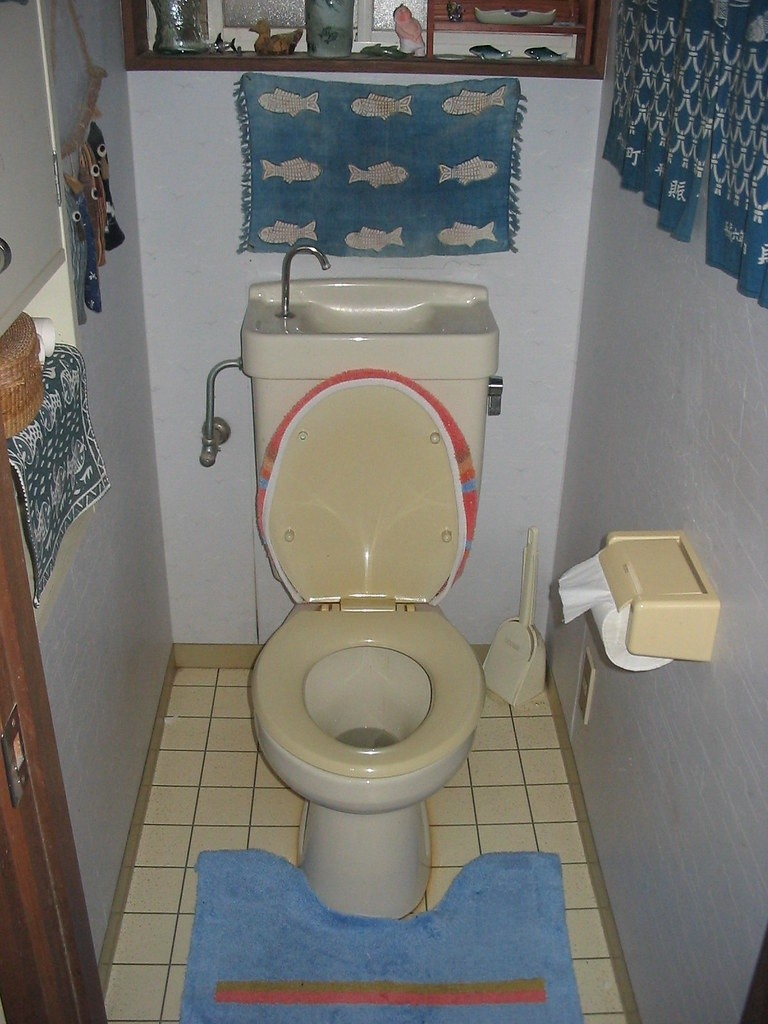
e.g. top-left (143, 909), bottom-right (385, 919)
top-left (557, 543), bottom-right (676, 674)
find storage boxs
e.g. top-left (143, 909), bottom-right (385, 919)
top-left (0, 311), bottom-right (47, 442)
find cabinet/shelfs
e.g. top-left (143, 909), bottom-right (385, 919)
top-left (427, 0), bottom-right (595, 66)
top-left (0, 0), bottom-right (69, 345)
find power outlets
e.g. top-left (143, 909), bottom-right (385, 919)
top-left (577, 644), bottom-right (597, 725)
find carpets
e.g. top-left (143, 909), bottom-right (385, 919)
top-left (177, 846), bottom-right (583, 1024)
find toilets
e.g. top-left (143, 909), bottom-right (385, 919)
top-left (236, 274), bottom-right (506, 921)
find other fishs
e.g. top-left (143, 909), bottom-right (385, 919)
top-left (524, 46), bottom-right (569, 62)
top-left (361, 43), bottom-right (415, 58)
top-left (468, 44), bottom-right (512, 60)
top-left (62, 119), bottom-right (125, 314)
top-left (210, 33), bottom-right (240, 55)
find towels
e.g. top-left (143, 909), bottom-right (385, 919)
top-left (5, 341), bottom-right (115, 612)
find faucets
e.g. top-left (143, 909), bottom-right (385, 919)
top-left (273, 243), bottom-right (333, 320)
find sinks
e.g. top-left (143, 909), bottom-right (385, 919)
top-left (238, 277), bottom-right (501, 382)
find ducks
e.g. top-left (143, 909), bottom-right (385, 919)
top-left (249, 20), bottom-right (303, 55)
